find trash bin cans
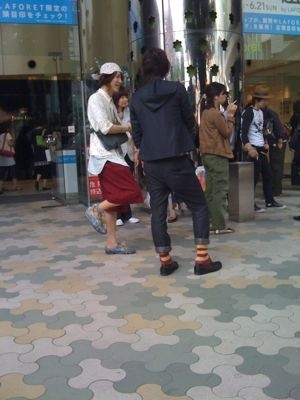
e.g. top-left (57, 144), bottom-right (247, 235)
top-left (228, 161), bottom-right (256, 222)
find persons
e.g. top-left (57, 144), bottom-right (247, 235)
top-left (85, 62), bottom-right (143, 254)
top-left (288, 114), bottom-right (300, 221)
top-left (112, 87), bottom-right (140, 226)
top-left (29, 118), bottom-right (53, 191)
top-left (240, 84), bottom-right (286, 212)
top-left (129, 48), bottom-right (222, 276)
top-left (0, 123), bottom-right (24, 194)
top-left (198, 82), bottom-right (238, 233)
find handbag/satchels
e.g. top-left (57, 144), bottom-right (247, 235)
top-left (35, 129), bottom-right (50, 150)
top-left (97, 121), bottom-right (128, 151)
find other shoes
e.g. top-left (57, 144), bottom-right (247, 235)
top-left (159, 261), bottom-right (179, 275)
top-left (168, 214), bottom-right (178, 223)
top-left (105, 242), bottom-right (136, 254)
top-left (254, 206), bottom-right (265, 213)
top-left (209, 227), bottom-right (233, 234)
top-left (41, 187), bottom-right (52, 190)
top-left (115, 216), bottom-right (141, 226)
top-left (194, 258), bottom-right (222, 275)
top-left (265, 201), bottom-right (283, 207)
top-left (35, 180), bottom-right (39, 191)
top-left (84, 206), bottom-right (107, 234)
top-left (293, 215), bottom-right (300, 221)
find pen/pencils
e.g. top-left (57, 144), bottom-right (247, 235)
top-left (227, 100), bottom-right (238, 109)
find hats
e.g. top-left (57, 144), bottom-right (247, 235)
top-left (99, 62), bottom-right (122, 75)
top-left (250, 84), bottom-right (275, 99)
top-left (142, 47), bottom-right (170, 78)
